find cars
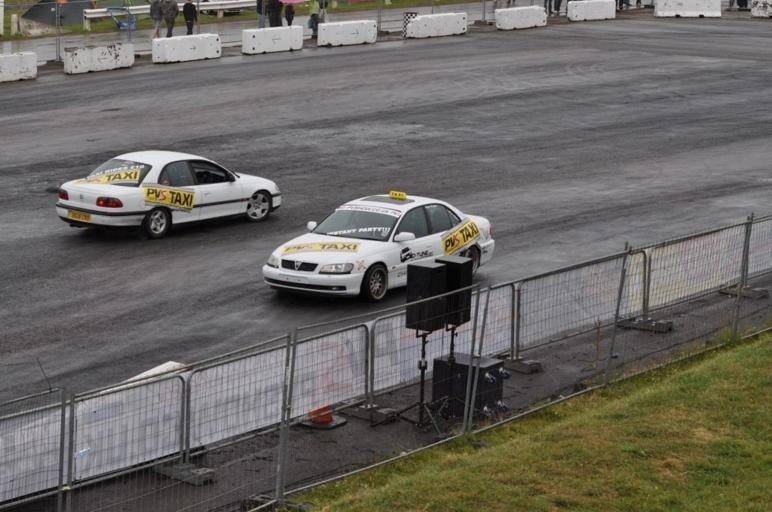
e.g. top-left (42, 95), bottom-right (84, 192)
top-left (262, 191), bottom-right (495, 302)
top-left (56, 150), bottom-right (282, 239)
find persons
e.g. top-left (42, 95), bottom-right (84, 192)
top-left (160, 0), bottom-right (179, 37)
top-left (505, 0), bottom-right (516, 5)
top-left (615, 0), bottom-right (624, 11)
top-left (266, 0), bottom-right (284, 27)
top-left (737, 0), bottom-right (748, 9)
top-left (553, 0), bottom-right (563, 14)
top-left (182, 0), bottom-right (197, 35)
top-left (148, 0), bottom-right (163, 41)
top-left (307, 11), bottom-right (322, 41)
top-left (256, 0), bottom-right (266, 28)
top-left (624, 1), bottom-right (641, 7)
top-left (285, 3), bottom-right (295, 26)
top-left (544, 0), bottom-right (553, 19)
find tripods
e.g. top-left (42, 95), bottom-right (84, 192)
top-left (370, 334), bottom-right (450, 441)
top-left (418, 330), bottom-right (489, 433)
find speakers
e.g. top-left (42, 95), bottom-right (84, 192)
top-left (435, 255), bottom-right (473, 326)
top-left (406, 261), bottom-right (447, 331)
